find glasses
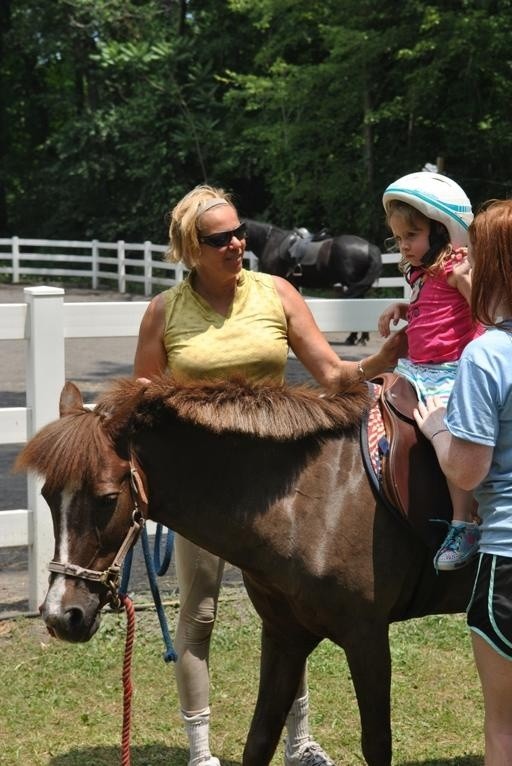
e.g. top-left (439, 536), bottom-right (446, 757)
top-left (197, 222), bottom-right (248, 247)
top-left (474, 198), bottom-right (500, 218)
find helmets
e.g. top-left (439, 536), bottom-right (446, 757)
top-left (382, 172), bottom-right (475, 252)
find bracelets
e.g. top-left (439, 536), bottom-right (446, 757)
top-left (357, 360), bottom-right (369, 382)
top-left (431, 429), bottom-right (449, 439)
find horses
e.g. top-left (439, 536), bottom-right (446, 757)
top-left (14, 369), bottom-right (468, 766)
top-left (240, 218), bottom-right (382, 346)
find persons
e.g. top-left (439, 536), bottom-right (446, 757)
top-left (412, 198), bottom-right (512, 766)
top-left (377, 170), bottom-right (487, 575)
top-left (132, 185), bottom-right (408, 765)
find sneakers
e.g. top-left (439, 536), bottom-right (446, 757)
top-left (427, 518), bottom-right (482, 577)
top-left (284, 735), bottom-right (335, 766)
